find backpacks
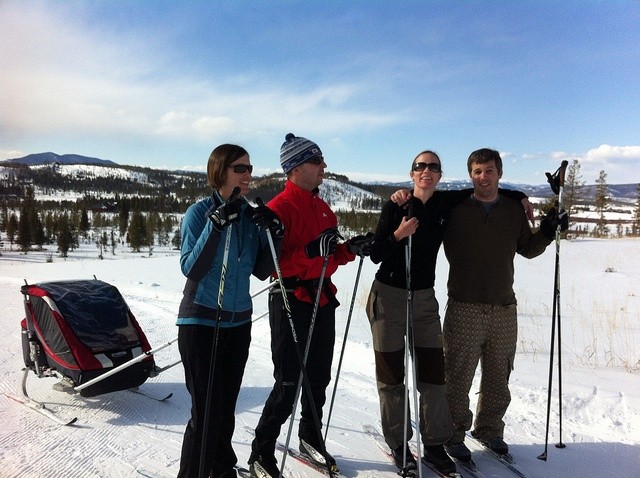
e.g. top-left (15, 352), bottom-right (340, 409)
top-left (2, 277), bottom-right (161, 400)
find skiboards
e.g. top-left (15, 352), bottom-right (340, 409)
top-left (233, 426), bottom-right (349, 478)
top-left (410, 420), bottom-right (529, 478)
top-left (363, 419), bottom-right (464, 478)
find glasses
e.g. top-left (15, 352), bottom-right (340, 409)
top-left (412, 162), bottom-right (441, 173)
top-left (306, 156), bottom-right (326, 165)
top-left (229, 163), bottom-right (254, 173)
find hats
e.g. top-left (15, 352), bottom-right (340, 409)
top-left (279, 132), bottom-right (322, 176)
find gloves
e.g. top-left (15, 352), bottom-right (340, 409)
top-left (346, 234), bottom-right (376, 258)
top-left (249, 196), bottom-right (286, 240)
top-left (540, 205), bottom-right (570, 239)
top-left (208, 186), bottom-right (244, 232)
top-left (305, 230), bottom-right (341, 260)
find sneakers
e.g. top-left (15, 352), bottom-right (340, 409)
top-left (299, 435), bottom-right (337, 468)
top-left (248, 458), bottom-right (286, 478)
top-left (423, 444), bottom-right (456, 475)
top-left (488, 439), bottom-right (510, 455)
top-left (446, 442), bottom-right (472, 461)
top-left (390, 444), bottom-right (417, 471)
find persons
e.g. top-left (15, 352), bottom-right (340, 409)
top-left (390, 148), bottom-right (568, 463)
top-left (365, 150), bottom-right (536, 475)
top-left (248, 133), bottom-right (376, 478)
top-left (174, 143), bottom-right (285, 478)
